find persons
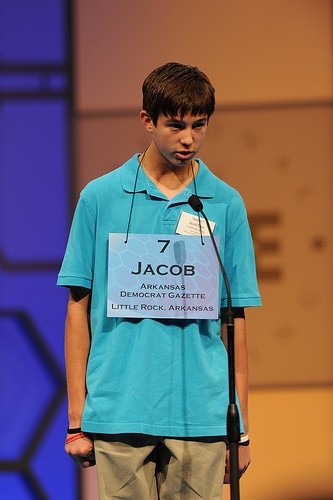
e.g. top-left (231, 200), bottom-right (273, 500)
top-left (56, 62), bottom-right (262, 500)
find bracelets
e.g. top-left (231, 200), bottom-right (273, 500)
top-left (67, 426), bottom-right (81, 434)
top-left (239, 435), bottom-right (249, 446)
top-left (65, 433), bottom-right (88, 444)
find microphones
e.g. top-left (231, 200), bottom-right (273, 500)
top-left (188, 195), bottom-right (231, 311)
